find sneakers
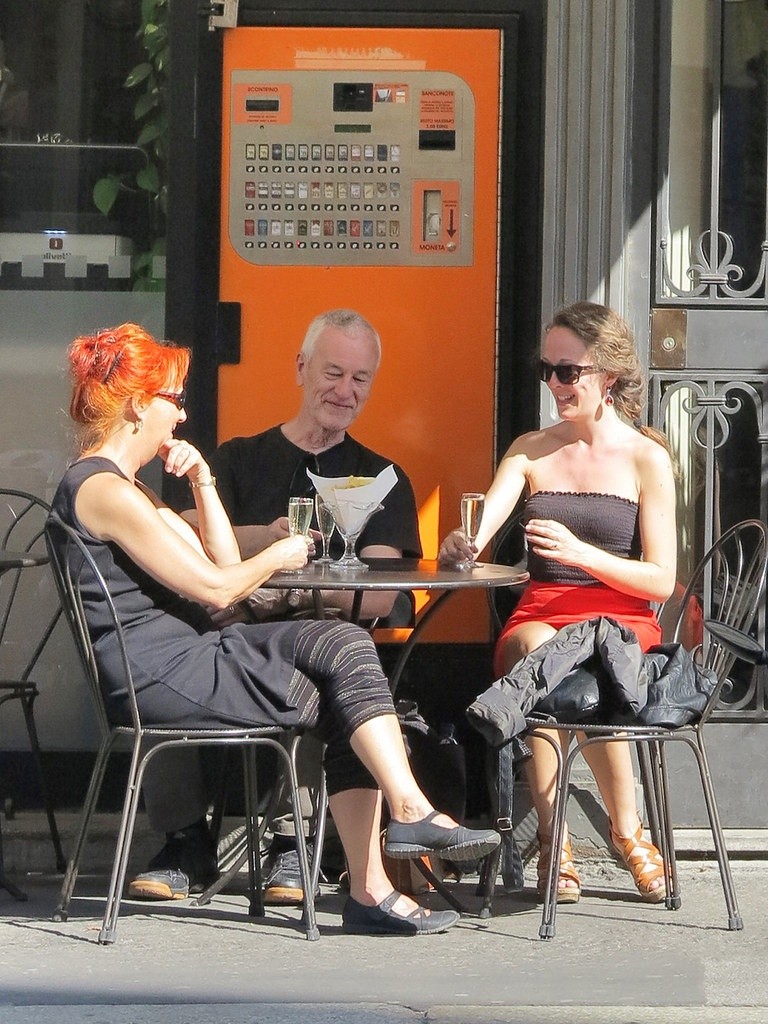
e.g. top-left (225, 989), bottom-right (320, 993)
top-left (128, 814), bottom-right (222, 900)
top-left (342, 891), bottom-right (461, 934)
top-left (385, 811), bottom-right (502, 861)
top-left (262, 837), bottom-right (321, 902)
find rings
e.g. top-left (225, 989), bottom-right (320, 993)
top-left (551, 540), bottom-right (558, 550)
top-left (229, 605), bottom-right (235, 616)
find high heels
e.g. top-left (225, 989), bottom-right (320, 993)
top-left (535, 827), bottom-right (580, 904)
top-left (607, 811), bottom-right (675, 903)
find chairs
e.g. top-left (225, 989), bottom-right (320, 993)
top-left (0, 486), bottom-right (768, 946)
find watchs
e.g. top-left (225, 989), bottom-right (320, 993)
top-left (285, 589), bottom-right (304, 612)
top-left (188, 476), bottom-right (216, 488)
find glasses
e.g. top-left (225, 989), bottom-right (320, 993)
top-left (156, 389), bottom-right (186, 411)
top-left (536, 360), bottom-right (606, 385)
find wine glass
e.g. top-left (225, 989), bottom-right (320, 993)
top-left (460, 493), bottom-right (485, 568)
top-left (324, 502), bottom-right (384, 569)
top-left (280, 496), bottom-right (314, 574)
top-left (315, 493), bottom-right (335, 564)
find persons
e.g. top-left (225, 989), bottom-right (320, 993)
top-left (44, 320), bottom-right (501, 938)
top-left (437, 299), bottom-right (686, 905)
top-left (128, 307), bottom-right (424, 904)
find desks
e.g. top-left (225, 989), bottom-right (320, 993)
top-left (222, 561), bottom-right (526, 931)
